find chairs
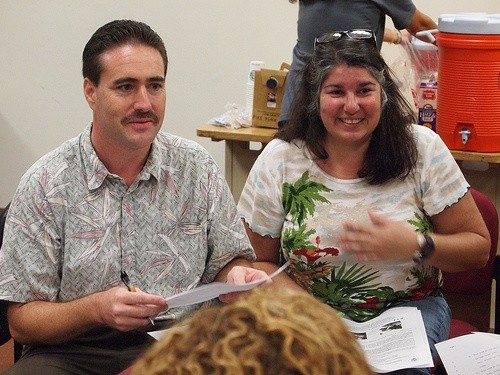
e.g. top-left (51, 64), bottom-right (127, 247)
top-left (442, 188), bottom-right (500, 339)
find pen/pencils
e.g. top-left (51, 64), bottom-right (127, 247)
top-left (120, 269), bottom-right (154, 325)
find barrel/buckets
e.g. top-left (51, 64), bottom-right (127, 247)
top-left (434, 12), bottom-right (500, 153)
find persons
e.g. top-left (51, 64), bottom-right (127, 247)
top-left (279, 0), bottom-right (437, 127)
top-left (236, 29), bottom-right (492, 375)
top-left (0, 20), bottom-right (274, 375)
top-left (133, 283), bottom-right (372, 375)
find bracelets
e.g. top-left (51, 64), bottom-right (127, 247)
top-left (413, 232), bottom-right (434, 265)
top-left (394, 29), bottom-right (402, 44)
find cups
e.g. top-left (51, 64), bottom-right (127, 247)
top-left (247, 60), bottom-right (265, 113)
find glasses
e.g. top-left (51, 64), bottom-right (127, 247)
top-left (312, 28), bottom-right (381, 51)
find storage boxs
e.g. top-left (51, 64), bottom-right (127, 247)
top-left (252, 69), bottom-right (288, 128)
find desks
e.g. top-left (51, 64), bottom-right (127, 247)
top-left (197, 122), bottom-right (500, 255)
